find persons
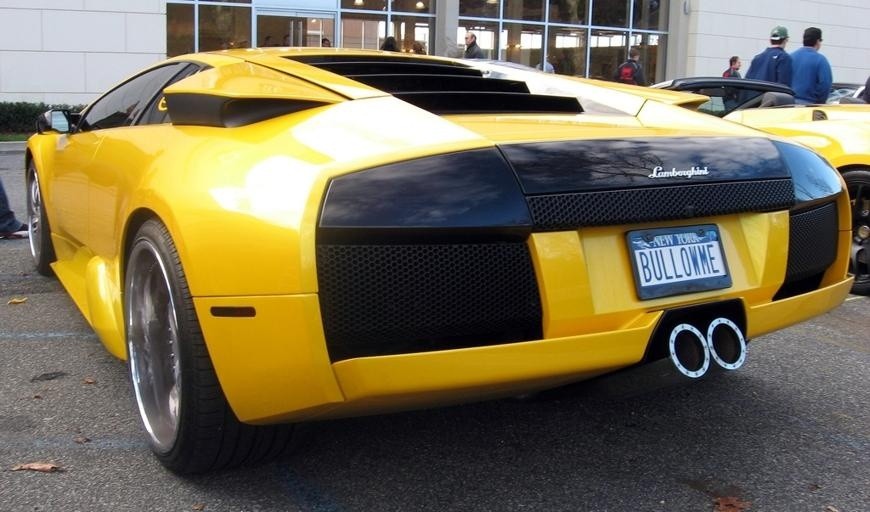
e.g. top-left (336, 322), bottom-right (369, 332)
top-left (261, 35), bottom-right (289, 46)
top-left (463, 32), bottom-right (484, 58)
top-left (380, 37), bottom-right (426, 54)
top-left (613, 47), bottom-right (646, 86)
top-left (722, 26), bottom-right (832, 111)
top-left (0, 180), bottom-right (28, 238)
top-left (536, 48), bottom-right (575, 73)
top-left (322, 38), bottom-right (331, 47)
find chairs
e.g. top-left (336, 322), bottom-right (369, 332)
top-left (757, 87), bottom-right (868, 113)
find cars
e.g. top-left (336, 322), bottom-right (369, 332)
top-left (717, 102), bottom-right (870, 296)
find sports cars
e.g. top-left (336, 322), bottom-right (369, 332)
top-left (25, 47), bottom-right (854, 477)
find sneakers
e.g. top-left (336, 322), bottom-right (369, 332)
top-left (3, 223), bottom-right (31, 241)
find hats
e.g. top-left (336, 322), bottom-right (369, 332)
top-left (769, 26), bottom-right (790, 41)
